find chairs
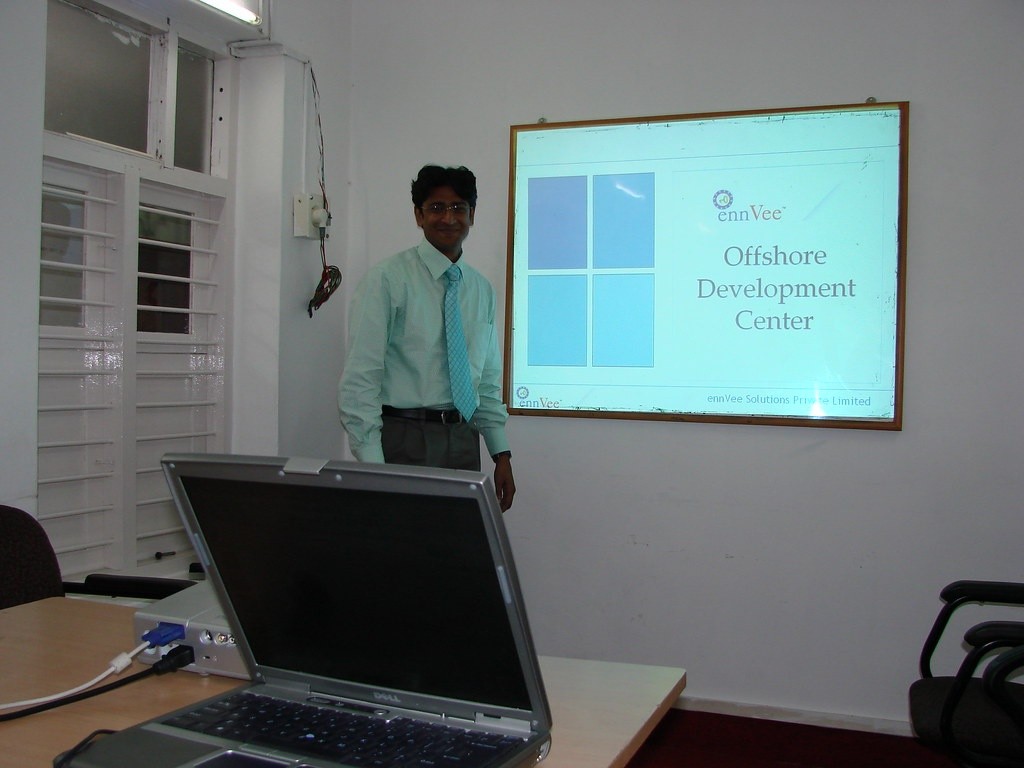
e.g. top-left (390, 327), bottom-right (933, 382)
top-left (908, 580), bottom-right (1024, 768)
top-left (0, 505), bottom-right (63, 614)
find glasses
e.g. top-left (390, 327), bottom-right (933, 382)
top-left (420, 204), bottom-right (471, 215)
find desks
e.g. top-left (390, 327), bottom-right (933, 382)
top-left (0, 596), bottom-right (690, 767)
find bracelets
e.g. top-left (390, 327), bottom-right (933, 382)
top-left (493, 451), bottom-right (511, 463)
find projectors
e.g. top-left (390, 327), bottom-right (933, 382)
top-left (133, 580), bottom-right (253, 681)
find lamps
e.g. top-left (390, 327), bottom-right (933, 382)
top-left (200, 0), bottom-right (262, 28)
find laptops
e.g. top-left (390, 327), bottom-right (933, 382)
top-left (52, 452), bottom-right (552, 768)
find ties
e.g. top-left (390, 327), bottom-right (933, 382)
top-left (444, 264), bottom-right (477, 422)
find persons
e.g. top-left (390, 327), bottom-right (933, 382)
top-left (338, 165), bottom-right (516, 514)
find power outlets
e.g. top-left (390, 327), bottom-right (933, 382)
top-left (294, 192), bottom-right (324, 240)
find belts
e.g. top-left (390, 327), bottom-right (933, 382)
top-left (383, 405), bottom-right (464, 425)
